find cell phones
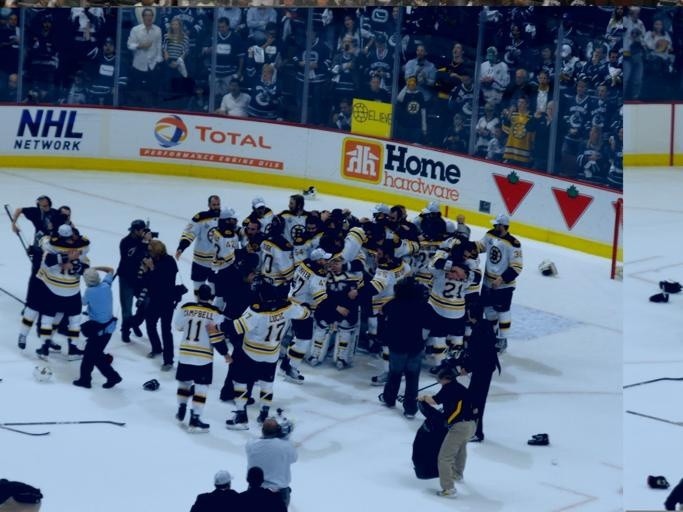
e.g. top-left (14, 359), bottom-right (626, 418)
top-left (344, 43), bottom-right (350, 51)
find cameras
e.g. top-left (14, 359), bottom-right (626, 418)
top-left (338, 110), bottom-right (345, 121)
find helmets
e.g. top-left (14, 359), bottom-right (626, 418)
top-left (58, 224), bottom-right (73, 237)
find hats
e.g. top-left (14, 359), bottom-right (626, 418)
top-left (369, 204), bottom-right (389, 214)
top-left (220, 207), bottom-right (239, 219)
top-left (252, 197), bottom-right (265, 209)
top-left (491, 214), bottom-right (509, 225)
top-left (215, 470), bottom-right (231, 485)
top-left (421, 201), bottom-right (440, 214)
top-left (311, 248), bottom-right (332, 261)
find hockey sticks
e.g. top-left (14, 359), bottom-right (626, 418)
top-left (5, 420), bottom-right (126, 427)
top-left (1, 426), bottom-right (49, 436)
top-left (622, 377), bottom-right (680, 388)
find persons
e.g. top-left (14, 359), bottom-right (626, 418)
top-left (12, 193), bottom-right (523, 512)
top-left (664, 479), bottom-right (683, 512)
top-left (0, 0), bottom-right (683, 190)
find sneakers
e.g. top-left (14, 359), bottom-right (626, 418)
top-left (372, 372), bottom-right (389, 382)
top-left (37, 339), bottom-right (61, 355)
top-left (176, 403), bottom-right (209, 428)
top-left (226, 410), bottom-right (248, 424)
top-left (103, 376), bottom-right (122, 387)
top-left (281, 354), bottom-right (304, 380)
top-left (74, 378), bottom-right (91, 388)
top-left (437, 488), bottom-right (458, 497)
top-left (68, 339), bottom-right (85, 355)
top-left (19, 334), bottom-right (26, 349)
top-left (257, 406), bottom-right (269, 421)
